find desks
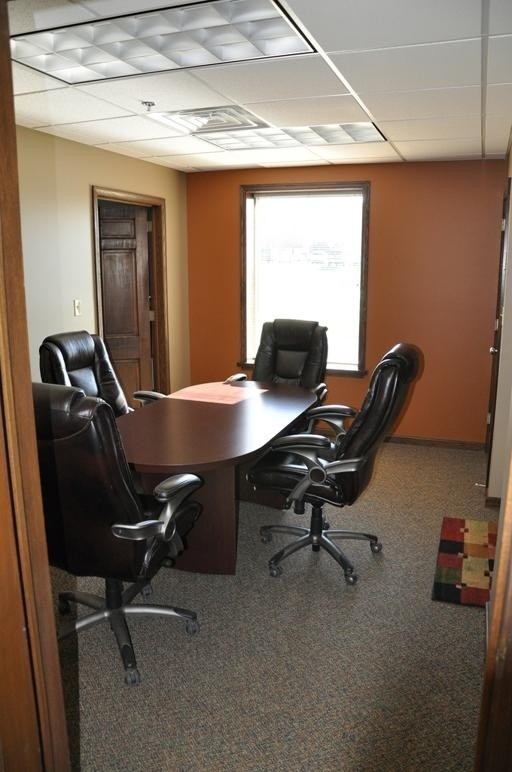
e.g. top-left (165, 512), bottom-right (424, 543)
top-left (116, 379), bottom-right (318, 575)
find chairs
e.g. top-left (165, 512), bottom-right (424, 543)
top-left (39, 330), bottom-right (166, 418)
top-left (235, 342), bottom-right (425, 586)
top-left (33, 381), bottom-right (203, 687)
top-left (227, 319), bottom-right (327, 511)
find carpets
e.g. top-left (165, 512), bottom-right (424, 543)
top-left (431, 517), bottom-right (498, 607)
